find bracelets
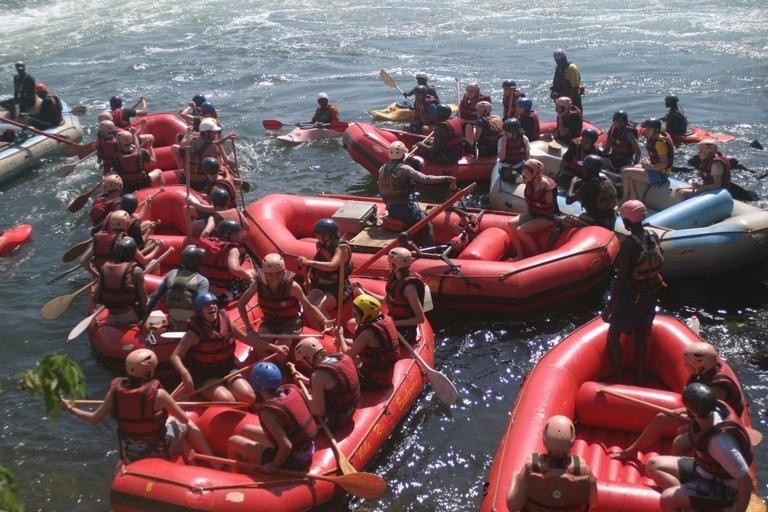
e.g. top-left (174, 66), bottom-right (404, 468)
top-left (69, 407), bottom-right (74, 414)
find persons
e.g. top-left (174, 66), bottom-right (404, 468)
top-left (3, 59), bottom-right (37, 121)
top-left (647, 384), bottom-right (757, 512)
top-left (611, 342), bottom-right (746, 459)
top-left (23, 83), bottom-right (63, 130)
top-left (296, 92), bottom-right (340, 129)
top-left (91, 94), bottom-right (253, 329)
top-left (60, 219), bottom-right (426, 468)
top-left (506, 415), bottom-right (598, 512)
top-left (599, 200), bottom-right (667, 378)
top-left (379, 50), bottom-right (732, 260)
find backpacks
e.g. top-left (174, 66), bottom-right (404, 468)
top-left (633, 234), bottom-right (663, 285)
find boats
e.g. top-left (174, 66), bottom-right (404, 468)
top-left (368, 99), bottom-right (460, 121)
top-left (87, 184), bottom-right (263, 366)
top-left (130, 110), bottom-right (190, 188)
top-left (242, 194), bottom-right (622, 300)
top-left (488, 140), bottom-right (768, 282)
top-left (628, 119), bottom-right (736, 145)
top-left (0, 84), bottom-right (83, 185)
top-left (478, 312), bottom-right (755, 512)
top-left (106, 277), bottom-right (437, 512)
top-left (0, 223), bottom-right (31, 257)
top-left (275, 121), bottom-right (355, 143)
top-left (341, 119), bottom-right (608, 184)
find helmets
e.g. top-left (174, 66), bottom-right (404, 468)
top-left (98, 93), bottom-right (242, 271)
top-left (697, 139), bottom-right (718, 160)
top-left (317, 92), bottom-right (329, 102)
top-left (261, 253), bottom-right (285, 273)
top-left (125, 293), bottom-right (382, 390)
top-left (314, 218), bottom-right (338, 234)
top-left (543, 415), bottom-right (576, 452)
top-left (389, 141), bottom-right (409, 159)
top-left (34, 83), bottom-right (47, 93)
top-left (613, 111), bottom-right (629, 126)
top-left (665, 93), bottom-right (677, 107)
top-left (15, 60), bottom-right (25, 70)
top-left (388, 247), bottom-right (412, 273)
top-left (681, 383), bottom-right (717, 416)
top-left (682, 340), bottom-right (719, 376)
top-left (415, 74), bottom-right (603, 180)
top-left (554, 49), bottom-right (565, 61)
top-left (620, 199), bottom-right (648, 224)
top-left (641, 117), bottom-right (662, 134)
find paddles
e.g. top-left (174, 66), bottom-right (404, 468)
top-left (373, 67), bottom-right (565, 160)
top-left (38, 94), bottom-right (177, 343)
top-left (158, 328), bottom-right (324, 340)
top-left (261, 121), bottom-right (348, 132)
top-left (220, 155), bottom-right (251, 193)
top-left (188, 449), bottom-right (386, 499)
top-left (352, 285), bottom-right (459, 407)
top-left (597, 387), bottom-right (762, 446)
top-left (18, 101), bottom-right (87, 118)
top-left (283, 361), bottom-right (359, 474)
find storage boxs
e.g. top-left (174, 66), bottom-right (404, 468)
top-left (332, 200), bottom-right (376, 235)
top-left (348, 214), bottom-right (403, 253)
